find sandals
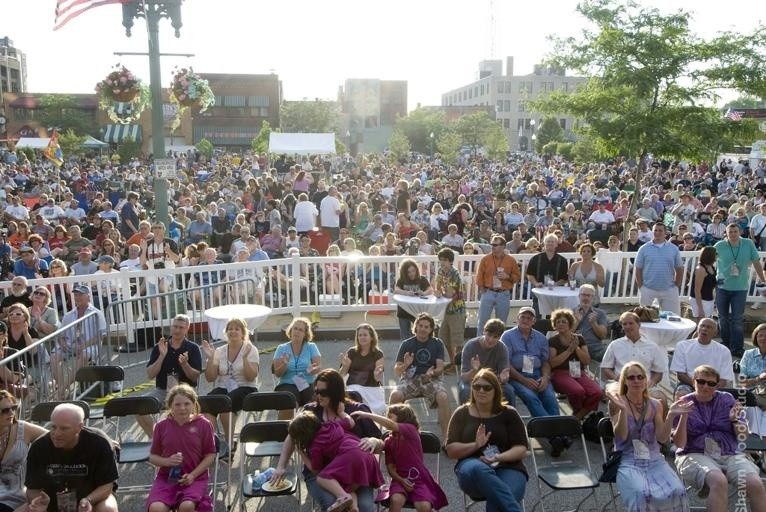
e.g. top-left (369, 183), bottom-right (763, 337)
top-left (327, 494), bottom-right (352, 512)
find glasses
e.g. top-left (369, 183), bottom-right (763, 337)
top-left (2, 406), bottom-right (17, 413)
top-left (472, 384), bottom-right (491, 391)
top-left (316, 389), bottom-right (329, 396)
top-left (627, 375), bottom-right (646, 380)
top-left (34, 291), bottom-right (44, 295)
top-left (696, 379), bottom-right (717, 386)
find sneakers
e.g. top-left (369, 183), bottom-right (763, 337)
top-left (551, 435), bottom-right (572, 456)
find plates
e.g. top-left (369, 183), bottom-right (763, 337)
top-left (261, 479), bottom-right (292, 493)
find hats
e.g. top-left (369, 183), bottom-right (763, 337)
top-left (519, 307), bottom-right (535, 316)
top-left (95, 256), bottom-right (114, 263)
top-left (72, 286), bottom-right (89, 293)
top-left (80, 248), bottom-right (89, 253)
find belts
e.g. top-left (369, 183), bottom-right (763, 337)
top-left (486, 287), bottom-right (505, 292)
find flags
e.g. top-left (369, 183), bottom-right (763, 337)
top-left (53, 1), bottom-right (135, 30)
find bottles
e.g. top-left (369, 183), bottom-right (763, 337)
top-left (651, 298), bottom-right (659, 309)
top-left (167, 453), bottom-right (182, 485)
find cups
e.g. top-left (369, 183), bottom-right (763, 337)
top-left (569, 281), bottom-right (575, 290)
top-left (547, 281), bottom-right (554, 291)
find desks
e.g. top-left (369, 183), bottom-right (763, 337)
top-left (640, 316), bottom-right (697, 352)
top-left (531, 284), bottom-right (581, 318)
top-left (204, 304), bottom-right (273, 344)
top-left (393, 293), bottom-right (454, 337)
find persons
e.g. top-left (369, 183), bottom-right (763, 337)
top-left (0, 149), bottom-right (766, 511)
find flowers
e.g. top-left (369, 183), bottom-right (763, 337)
top-left (94, 63), bottom-right (153, 124)
top-left (169, 66), bottom-right (215, 133)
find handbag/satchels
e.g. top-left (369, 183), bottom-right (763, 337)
top-left (598, 451), bottom-right (623, 482)
top-left (583, 411), bottom-right (613, 444)
